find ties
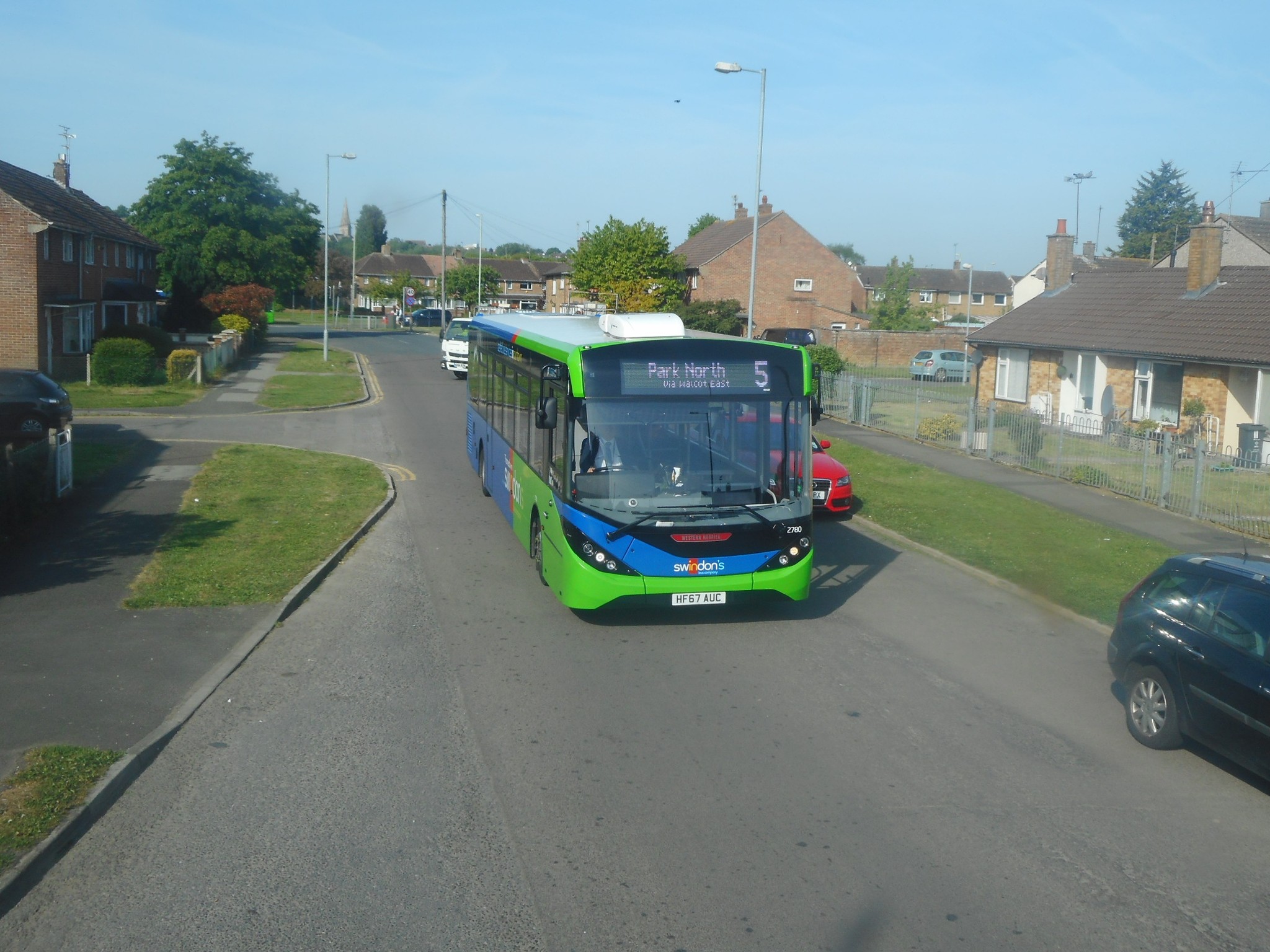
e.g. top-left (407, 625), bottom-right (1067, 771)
top-left (605, 441), bottom-right (613, 472)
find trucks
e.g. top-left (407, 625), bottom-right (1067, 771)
top-left (438, 317), bottom-right (482, 380)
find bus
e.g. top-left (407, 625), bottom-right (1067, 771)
top-left (464, 310), bottom-right (823, 613)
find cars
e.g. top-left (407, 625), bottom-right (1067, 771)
top-left (716, 411), bottom-right (852, 516)
top-left (399, 309), bottom-right (453, 327)
top-left (908, 350), bottom-right (972, 383)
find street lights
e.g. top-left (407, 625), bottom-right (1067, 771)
top-left (712, 59), bottom-right (766, 340)
top-left (961, 263), bottom-right (974, 387)
top-left (323, 153), bottom-right (358, 361)
top-left (475, 213), bottom-right (482, 313)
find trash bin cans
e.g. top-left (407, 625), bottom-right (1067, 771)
top-left (1233, 423), bottom-right (1267, 469)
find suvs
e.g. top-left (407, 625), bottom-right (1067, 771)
top-left (1108, 553), bottom-right (1270, 783)
top-left (0, 367), bottom-right (73, 439)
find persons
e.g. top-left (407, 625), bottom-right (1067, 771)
top-left (579, 413), bottom-right (635, 474)
top-left (392, 304), bottom-right (404, 328)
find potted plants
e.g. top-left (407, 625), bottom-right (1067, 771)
top-left (1111, 421), bottom-right (1162, 453)
top-left (1213, 460), bottom-right (1235, 472)
